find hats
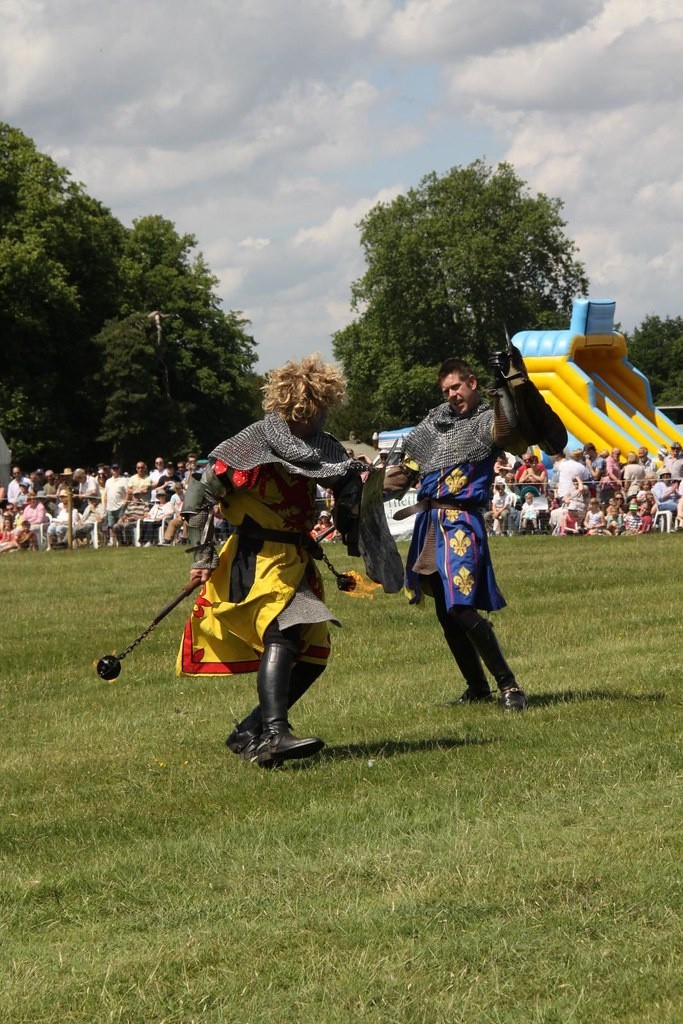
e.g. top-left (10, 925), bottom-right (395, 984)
top-left (156, 489), bottom-right (168, 498)
top-left (659, 468), bottom-right (671, 476)
top-left (19, 482), bottom-right (30, 488)
top-left (319, 510), bottom-right (330, 519)
top-left (567, 503), bottom-right (581, 511)
top-left (165, 461), bottom-right (174, 468)
top-left (59, 489), bottom-right (68, 496)
top-left (60, 468), bottom-right (73, 475)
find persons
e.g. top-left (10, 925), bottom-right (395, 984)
top-left (179, 352), bottom-right (364, 769)
top-left (0, 442), bottom-right (683, 555)
top-left (378, 349), bottom-right (529, 713)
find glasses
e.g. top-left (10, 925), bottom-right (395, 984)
top-left (671, 446), bottom-right (682, 451)
top-left (111, 467), bottom-right (119, 470)
top-left (616, 498), bottom-right (623, 501)
top-left (37, 473), bottom-right (44, 476)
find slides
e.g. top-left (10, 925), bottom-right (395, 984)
top-left (569, 344), bottom-right (683, 467)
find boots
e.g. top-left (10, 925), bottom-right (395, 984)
top-left (226, 660), bottom-right (327, 768)
top-left (250, 643), bottom-right (325, 767)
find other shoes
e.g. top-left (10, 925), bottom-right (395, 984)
top-left (521, 529), bottom-right (526, 535)
top-left (108, 541), bottom-right (114, 546)
top-left (501, 530), bottom-right (507, 536)
top-left (54, 542), bottom-right (67, 548)
top-left (451, 682), bottom-right (492, 707)
top-left (143, 541), bottom-right (151, 547)
top-left (157, 540), bottom-right (171, 547)
top-left (46, 543), bottom-right (53, 552)
top-left (134, 541), bottom-right (141, 547)
top-left (501, 684), bottom-right (525, 713)
top-left (489, 531), bottom-right (496, 536)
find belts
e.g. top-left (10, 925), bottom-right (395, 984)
top-left (392, 496), bottom-right (487, 520)
top-left (232, 522), bottom-right (312, 547)
